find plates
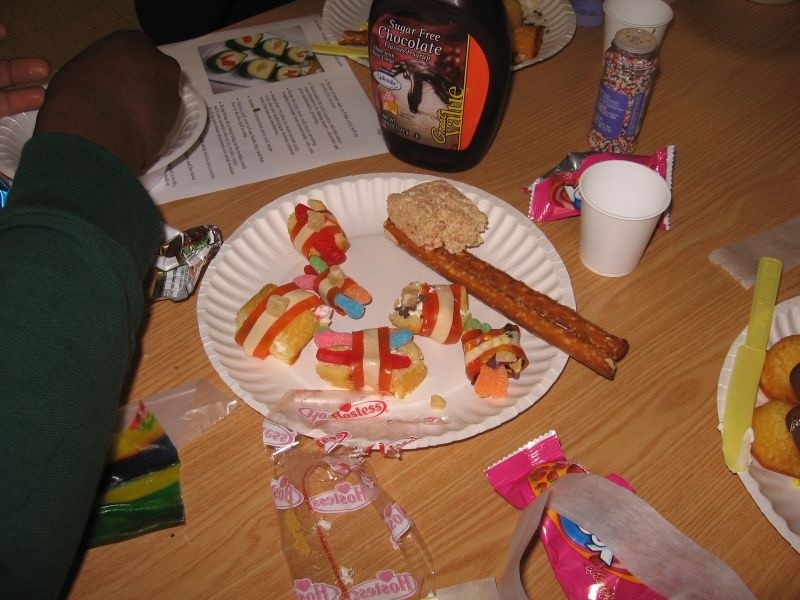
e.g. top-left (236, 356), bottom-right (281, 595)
top-left (201, 30), bottom-right (309, 87)
top-left (321, 0), bottom-right (580, 73)
top-left (0, 78), bottom-right (208, 178)
top-left (715, 292), bottom-right (800, 554)
top-left (195, 171), bottom-right (576, 452)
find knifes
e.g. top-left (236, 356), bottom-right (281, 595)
top-left (723, 257), bottom-right (783, 471)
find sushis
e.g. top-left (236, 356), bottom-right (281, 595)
top-left (206, 32), bottom-right (310, 82)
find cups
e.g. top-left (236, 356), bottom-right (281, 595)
top-left (603, 0), bottom-right (673, 70)
top-left (578, 160), bottom-right (671, 278)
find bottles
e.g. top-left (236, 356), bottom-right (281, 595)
top-left (588, 28), bottom-right (663, 155)
top-left (366, 0), bottom-right (514, 172)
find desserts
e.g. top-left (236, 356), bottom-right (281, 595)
top-left (234, 180), bottom-right (630, 402)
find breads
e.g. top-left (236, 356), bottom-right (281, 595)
top-left (506, 0), bottom-right (544, 60)
top-left (750, 335), bottom-right (800, 480)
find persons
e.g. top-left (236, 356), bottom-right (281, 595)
top-left (0, 30), bottom-right (184, 600)
